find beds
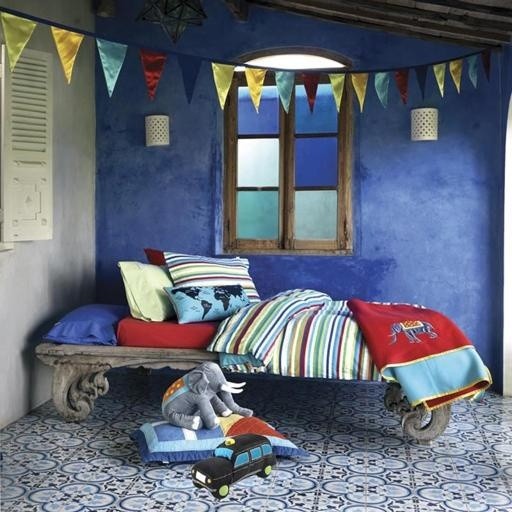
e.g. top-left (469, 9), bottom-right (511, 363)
top-left (34, 299), bottom-right (454, 441)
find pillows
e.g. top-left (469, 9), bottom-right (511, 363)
top-left (116, 247), bottom-right (262, 325)
top-left (128, 411), bottom-right (310, 465)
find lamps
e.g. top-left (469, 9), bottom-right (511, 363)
top-left (236, 46), bottom-right (353, 74)
top-left (409, 107), bottom-right (438, 142)
top-left (143, 114), bottom-right (170, 148)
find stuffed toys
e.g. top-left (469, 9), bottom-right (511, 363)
top-left (161, 361), bottom-right (254, 431)
